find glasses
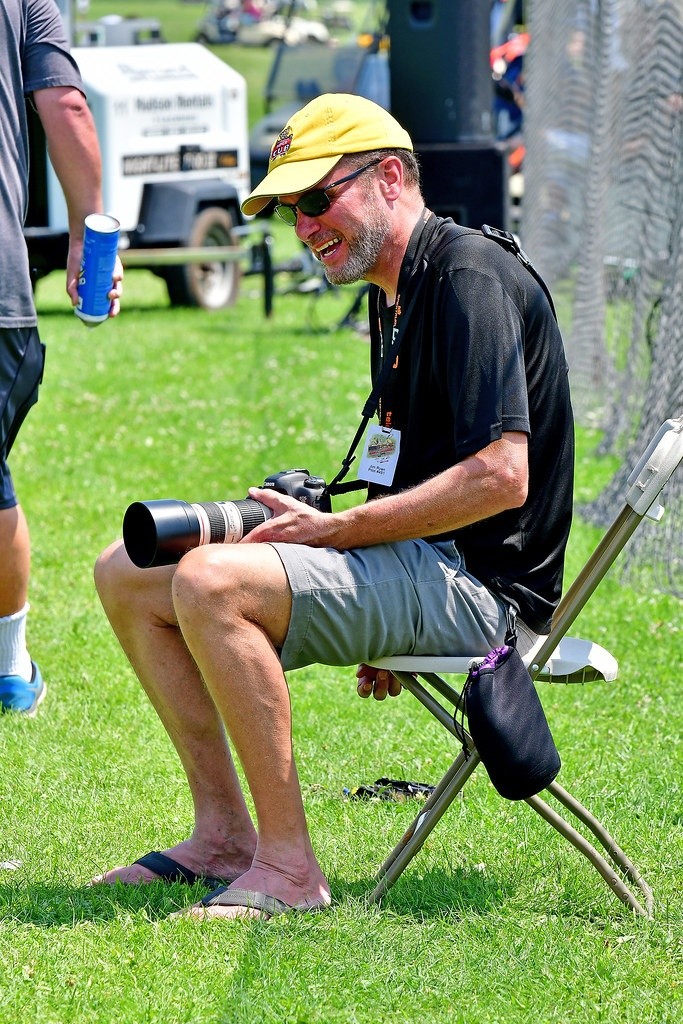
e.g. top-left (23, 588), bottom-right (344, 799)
top-left (273, 160), bottom-right (381, 227)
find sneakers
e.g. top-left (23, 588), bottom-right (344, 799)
top-left (0, 661), bottom-right (47, 719)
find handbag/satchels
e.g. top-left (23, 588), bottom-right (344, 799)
top-left (454, 606), bottom-right (561, 802)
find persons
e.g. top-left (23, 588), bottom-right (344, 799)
top-left (0, 1), bottom-right (125, 718)
top-left (93, 93), bottom-right (575, 922)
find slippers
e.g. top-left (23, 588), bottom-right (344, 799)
top-left (167, 886), bottom-right (330, 928)
top-left (93, 850), bottom-right (229, 888)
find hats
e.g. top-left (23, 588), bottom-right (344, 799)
top-left (240, 93), bottom-right (414, 216)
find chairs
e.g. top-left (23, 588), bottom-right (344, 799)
top-left (356, 416), bottom-right (683, 927)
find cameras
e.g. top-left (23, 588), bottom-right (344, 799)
top-left (122, 468), bottom-right (333, 569)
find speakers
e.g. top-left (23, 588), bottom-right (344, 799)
top-left (387, 0), bottom-right (495, 144)
top-left (412, 144), bottom-right (512, 231)
